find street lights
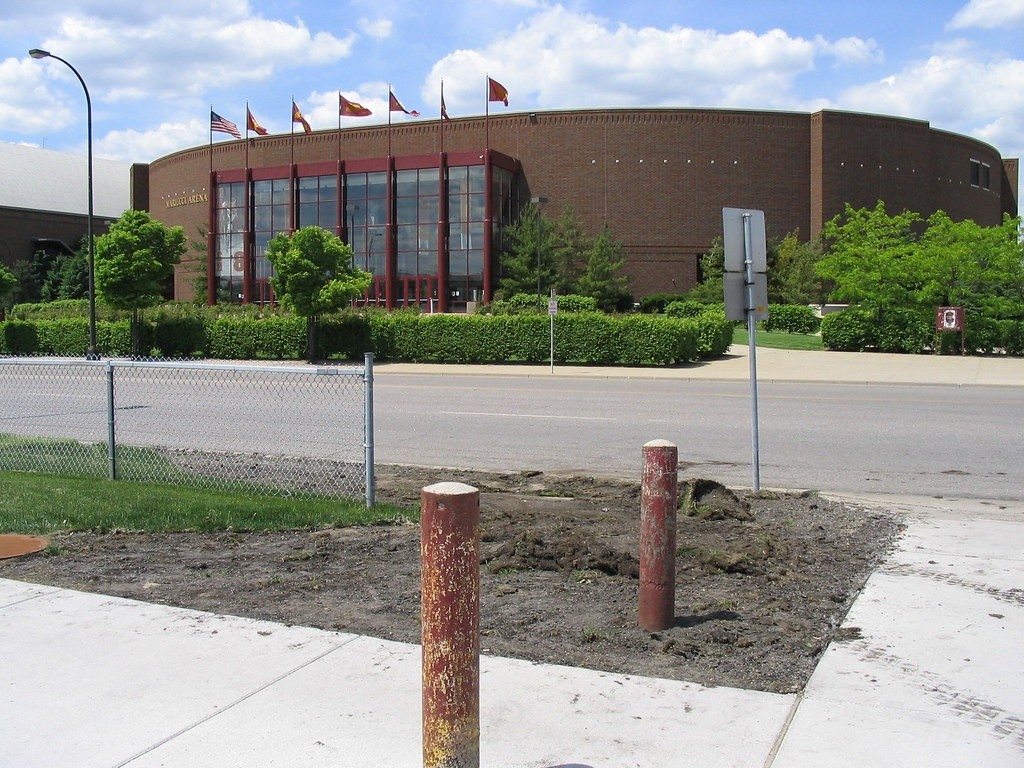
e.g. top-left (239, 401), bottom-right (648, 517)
top-left (531, 197), bottom-right (547, 312)
top-left (28, 49), bottom-right (99, 359)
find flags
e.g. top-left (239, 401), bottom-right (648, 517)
top-left (390, 92), bottom-right (420, 116)
top-left (247, 110), bottom-right (268, 135)
top-left (442, 90), bottom-right (451, 123)
top-left (340, 96), bottom-right (373, 116)
top-left (293, 103), bottom-right (311, 134)
top-left (211, 111), bottom-right (241, 139)
top-left (489, 78), bottom-right (508, 107)
